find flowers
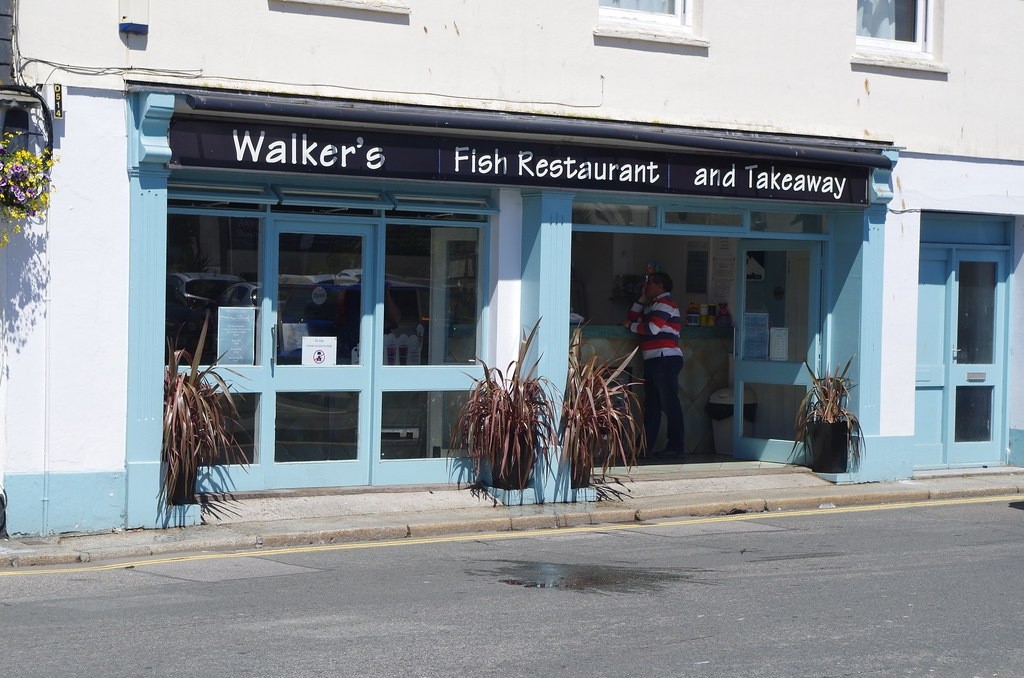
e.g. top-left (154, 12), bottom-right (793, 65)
top-left (0, 129), bottom-right (60, 251)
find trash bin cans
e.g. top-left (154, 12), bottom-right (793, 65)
top-left (708, 386), bottom-right (758, 455)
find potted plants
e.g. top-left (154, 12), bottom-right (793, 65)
top-left (560, 317), bottom-right (649, 490)
top-left (447, 316), bottom-right (563, 490)
top-left (165, 309), bottom-right (252, 506)
top-left (787, 354), bottom-right (866, 474)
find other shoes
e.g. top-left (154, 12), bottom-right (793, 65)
top-left (621, 445), bottom-right (650, 459)
top-left (647, 442), bottom-right (685, 460)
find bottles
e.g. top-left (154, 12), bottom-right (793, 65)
top-left (645, 263), bottom-right (661, 273)
top-left (685, 299), bottom-right (731, 328)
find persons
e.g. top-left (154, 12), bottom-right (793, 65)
top-left (618, 270), bottom-right (686, 458)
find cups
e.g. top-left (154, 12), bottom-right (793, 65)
top-left (386, 343), bottom-right (419, 365)
